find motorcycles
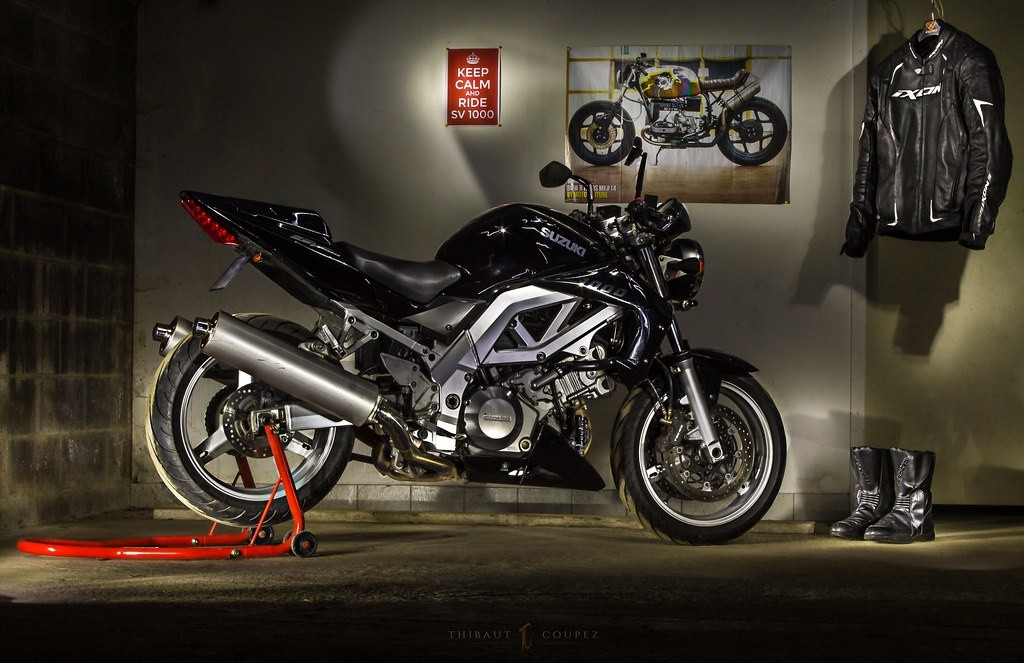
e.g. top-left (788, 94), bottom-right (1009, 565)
top-left (143, 153), bottom-right (787, 545)
top-left (568, 53), bottom-right (790, 167)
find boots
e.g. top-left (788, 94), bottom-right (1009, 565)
top-left (864, 446), bottom-right (937, 544)
top-left (829, 446), bottom-right (896, 539)
